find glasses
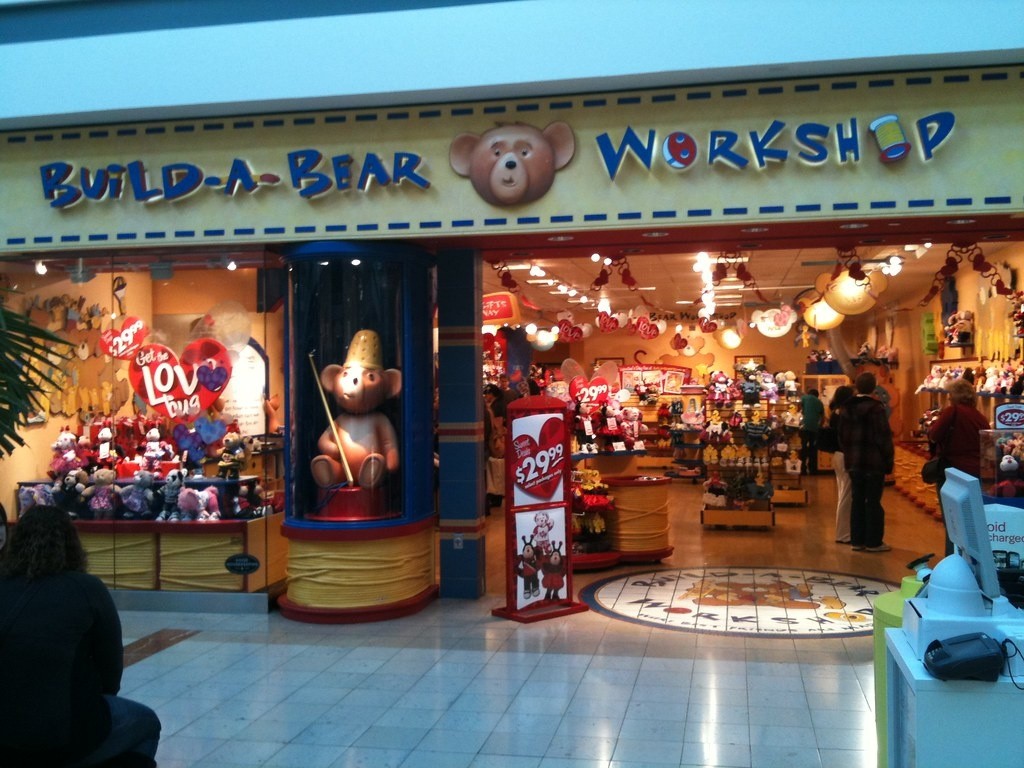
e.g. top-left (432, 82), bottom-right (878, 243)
top-left (483, 389), bottom-right (494, 394)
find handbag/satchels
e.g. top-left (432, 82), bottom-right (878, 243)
top-left (848, 444), bottom-right (888, 481)
top-left (921, 459), bottom-right (947, 484)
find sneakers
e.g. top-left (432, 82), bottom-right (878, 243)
top-left (850, 543), bottom-right (892, 552)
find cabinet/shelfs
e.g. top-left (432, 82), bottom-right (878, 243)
top-left (700, 395), bottom-right (776, 532)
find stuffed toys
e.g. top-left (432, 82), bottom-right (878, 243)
top-left (910, 293), bottom-right (1024, 497)
top-left (311, 328), bottom-right (402, 489)
top-left (17, 414), bottom-right (285, 522)
top-left (570, 358), bottom-right (802, 555)
top-left (804, 341), bottom-right (898, 363)
top-left (482, 332), bottom-right (556, 392)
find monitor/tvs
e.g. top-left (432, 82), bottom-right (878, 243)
top-left (941, 467), bottom-right (1001, 600)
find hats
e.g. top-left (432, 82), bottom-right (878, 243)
top-left (828, 386), bottom-right (855, 409)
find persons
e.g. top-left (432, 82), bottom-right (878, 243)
top-left (829, 372), bottom-right (895, 553)
top-left (482, 361), bottom-right (556, 519)
top-left (929, 379), bottom-right (992, 557)
top-left (797, 387), bottom-right (826, 476)
top-left (0, 502), bottom-right (161, 767)
